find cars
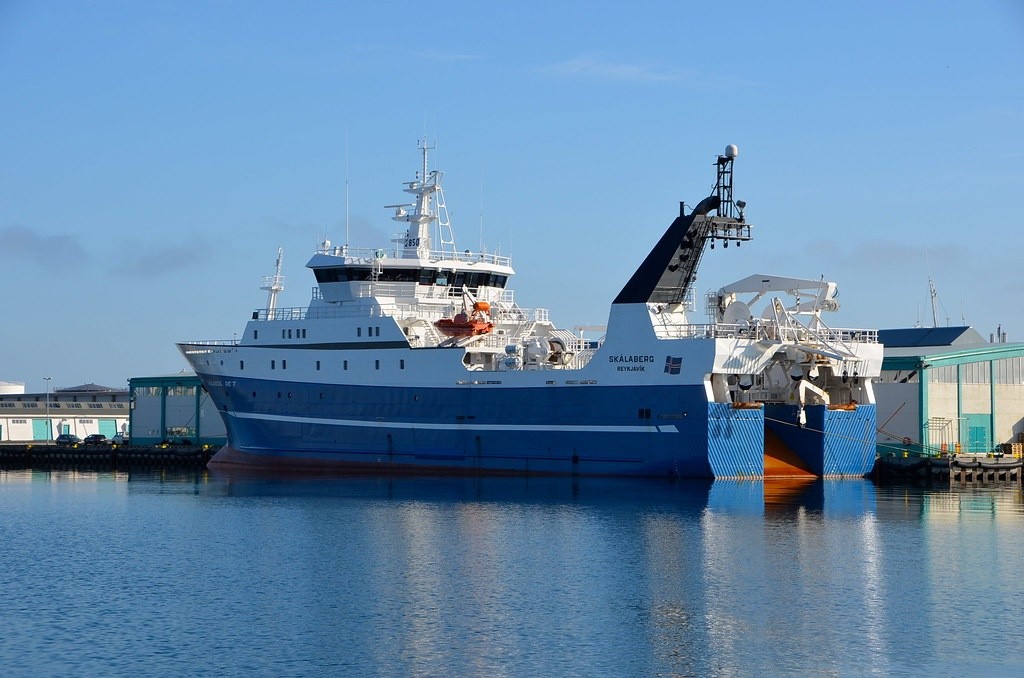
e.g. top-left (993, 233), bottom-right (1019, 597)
top-left (55, 434), bottom-right (85, 447)
top-left (112, 432), bottom-right (129, 445)
top-left (84, 434), bottom-right (107, 445)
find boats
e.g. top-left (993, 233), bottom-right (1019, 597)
top-left (173, 136), bottom-right (885, 482)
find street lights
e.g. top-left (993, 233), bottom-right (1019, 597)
top-left (43, 377), bottom-right (51, 445)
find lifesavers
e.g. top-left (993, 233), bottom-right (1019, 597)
top-left (903, 437), bottom-right (910, 445)
